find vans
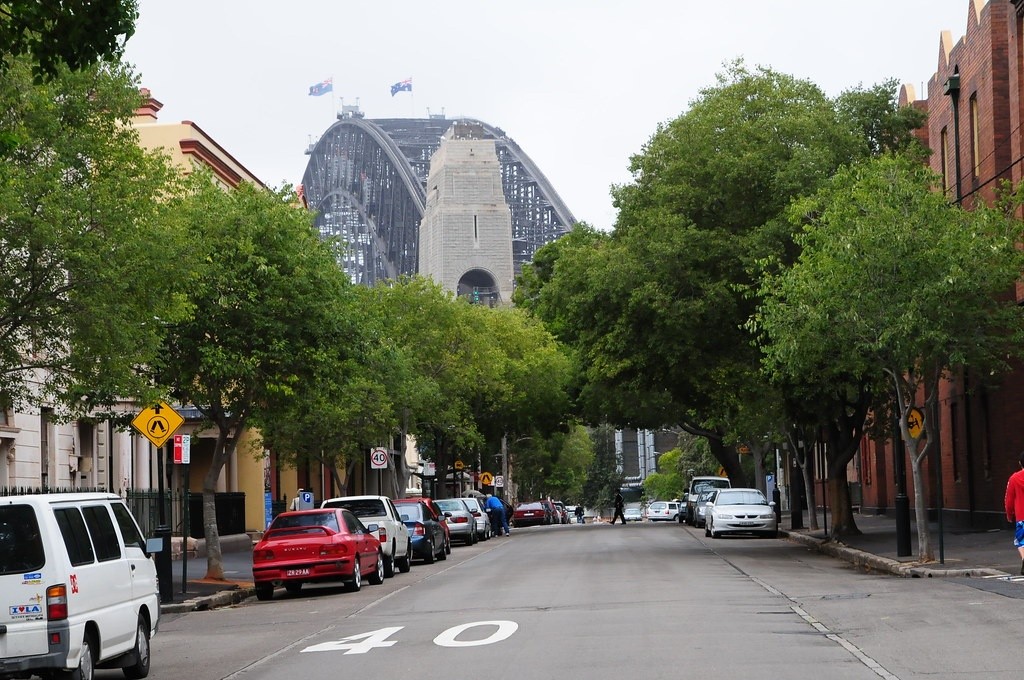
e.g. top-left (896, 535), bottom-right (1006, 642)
top-left (680, 475), bottom-right (732, 524)
top-left (0, 491), bottom-right (162, 680)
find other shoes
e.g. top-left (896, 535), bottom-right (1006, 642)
top-left (1021, 559), bottom-right (1024, 575)
top-left (621, 522), bottom-right (626, 524)
top-left (506, 533), bottom-right (509, 537)
top-left (495, 534), bottom-right (498, 538)
top-left (609, 521), bottom-right (614, 524)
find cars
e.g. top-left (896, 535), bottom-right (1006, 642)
top-left (512, 499), bottom-right (571, 526)
top-left (645, 501), bottom-right (681, 521)
top-left (622, 508), bottom-right (643, 521)
top-left (251, 493), bottom-right (494, 602)
top-left (693, 488), bottom-right (782, 539)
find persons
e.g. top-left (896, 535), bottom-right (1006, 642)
top-left (289, 488), bottom-right (304, 511)
top-left (609, 488), bottom-right (626, 525)
top-left (575, 504), bottom-right (584, 523)
top-left (1005, 453), bottom-right (1024, 576)
top-left (486, 494), bottom-right (510, 537)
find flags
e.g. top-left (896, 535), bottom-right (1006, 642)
top-left (390, 77), bottom-right (413, 96)
top-left (309, 77), bottom-right (332, 96)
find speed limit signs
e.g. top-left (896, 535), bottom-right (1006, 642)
top-left (371, 450), bottom-right (387, 465)
